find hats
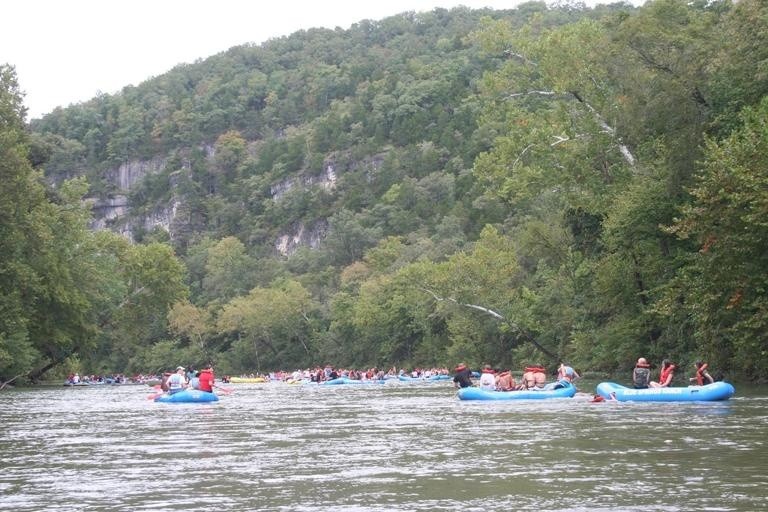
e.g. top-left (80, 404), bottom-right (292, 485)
top-left (525, 364), bottom-right (545, 371)
top-left (455, 363), bottom-right (466, 370)
top-left (176, 365), bottom-right (185, 370)
top-left (566, 367), bottom-right (573, 375)
top-left (482, 365), bottom-right (494, 373)
top-left (636, 357), bottom-right (650, 368)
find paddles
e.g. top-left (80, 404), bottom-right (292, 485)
top-left (211, 385), bottom-right (232, 393)
top-left (147, 393), bottom-right (166, 400)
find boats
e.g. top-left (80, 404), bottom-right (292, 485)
top-left (156, 387), bottom-right (220, 403)
top-left (455, 381), bottom-right (577, 400)
top-left (596, 380), bottom-right (736, 404)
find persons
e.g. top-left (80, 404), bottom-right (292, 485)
top-left (241, 364), bottom-right (450, 383)
top-left (588, 392), bottom-right (618, 404)
top-left (453, 361), bottom-right (580, 391)
top-left (161, 363), bottom-right (217, 395)
top-left (633, 357), bottom-right (714, 389)
top-left (65, 372), bottom-right (161, 384)
